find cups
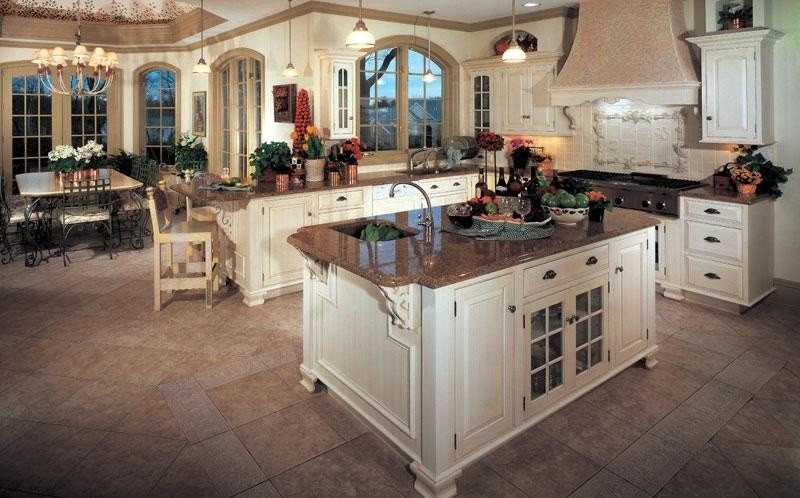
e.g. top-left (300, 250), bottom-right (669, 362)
top-left (99, 168), bottom-right (112, 179)
top-left (199, 175), bottom-right (219, 188)
top-left (220, 168), bottom-right (229, 178)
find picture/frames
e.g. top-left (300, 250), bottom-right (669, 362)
top-left (192, 91), bottom-right (207, 137)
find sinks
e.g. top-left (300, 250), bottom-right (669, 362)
top-left (329, 219), bottom-right (420, 242)
top-left (397, 168), bottom-right (446, 175)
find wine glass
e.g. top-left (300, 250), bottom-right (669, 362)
top-left (500, 198), bottom-right (513, 232)
top-left (511, 196), bottom-right (532, 233)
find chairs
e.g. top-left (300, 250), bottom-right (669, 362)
top-left (0, 158), bottom-right (156, 266)
top-left (147, 181), bottom-right (220, 311)
top-left (185, 169), bottom-right (217, 224)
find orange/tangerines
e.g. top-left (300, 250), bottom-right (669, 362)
top-left (541, 189), bottom-right (590, 209)
top-left (231, 177), bottom-right (241, 185)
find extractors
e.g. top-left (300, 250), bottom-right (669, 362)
top-left (551, 0), bottom-right (702, 107)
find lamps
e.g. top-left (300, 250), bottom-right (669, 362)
top-left (420, 69), bottom-right (439, 83)
top-left (279, 61), bottom-right (303, 81)
top-left (32, 0), bottom-right (119, 95)
top-left (193, 56), bottom-right (213, 77)
top-left (501, 42), bottom-right (528, 65)
top-left (342, 21), bottom-right (378, 51)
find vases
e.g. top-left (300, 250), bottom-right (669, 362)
top-left (727, 18), bottom-right (743, 29)
top-left (589, 203), bottom-right (604, 222)
top-left (276, 159), bottom-right (357, 194)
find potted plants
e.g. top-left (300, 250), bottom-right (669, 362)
top-left (250, 141), bottom-right (292, 182)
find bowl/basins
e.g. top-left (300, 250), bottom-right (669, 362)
top-left (447, 214), bottom-right (472, 227)
top-left (542, 205), bottom-right (590, 226)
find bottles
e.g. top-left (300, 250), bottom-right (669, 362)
top-left (551, 169), bottom-right (560, 183)
top-left (475, 168), bottom-right (488, 199)
top-left (528, 166), bottom-right (546, 193)
top-left (495, 166), bottom-right (508, 196)
top-left (532, 358), bottom-right (555, 393)
top-left (507, 168), bottom-right (518, 195)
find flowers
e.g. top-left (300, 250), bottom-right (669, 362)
top-left (587, 191), bottom-right (613, 212)
top-left (290, 127), bottom-right (362, 158)
top-left (717, 0), bottom-right (744, 24)
top-left (48, 140), bottom-right (106, 172)
top-left (169, 130), bottom-right (207, 172)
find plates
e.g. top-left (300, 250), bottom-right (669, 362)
top-left (447, 136), bottom-right (479, 159)
top-left (213, 182), bottom-right (250, 192)
top-left (542, 310), bottom-right (561, 318)
top-left (473, 213), bottom-right (552, 226)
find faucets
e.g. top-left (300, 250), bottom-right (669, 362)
top-left (408, 146), bottom-right (427, 171)
top-left (423, 150), bottom-right (439, 169)
top-left (390, 181), bottom-right (434, 242)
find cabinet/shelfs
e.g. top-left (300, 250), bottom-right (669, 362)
top-left (685, 26), bottom-right (785, 146)
top-left (679, 196), bottom-right (776, 314)
top-left (448, 228), bottom-right (656, 470)
top-left (315, 49), bottom-right (366, 140)
top-left (462, 53), bottom-right (581, 168)
top-left (251, 176), bottom-right (469, 305)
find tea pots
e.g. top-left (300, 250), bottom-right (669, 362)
top-left (434, 146), bottom-right (457, 171)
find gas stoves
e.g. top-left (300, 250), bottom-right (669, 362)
top-left (561, 168), bottom-right (700, 219)
